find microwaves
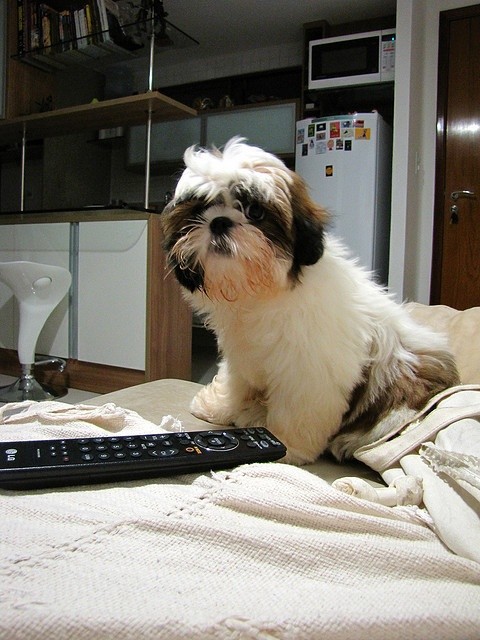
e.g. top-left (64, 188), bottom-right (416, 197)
top-left (307, 27), bottom-right (396, 90)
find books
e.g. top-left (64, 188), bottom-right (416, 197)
top-left (14, 1), bottom-right (133, 74)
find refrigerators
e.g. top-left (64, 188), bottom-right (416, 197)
top-left (295, 108), bottom-right (392, 288)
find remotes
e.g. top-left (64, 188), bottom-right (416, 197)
top-left (0, 425), bottom-right (288, 490)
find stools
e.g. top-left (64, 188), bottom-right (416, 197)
top-left (0, 261), bottom-right (71, 403)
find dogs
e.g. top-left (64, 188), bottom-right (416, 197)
top-left (160, 134), bottom-right (460, 468)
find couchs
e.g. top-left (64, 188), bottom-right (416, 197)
top-left (0, 303), bottom-right (480, 639)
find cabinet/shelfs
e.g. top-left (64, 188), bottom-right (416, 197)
top-left (2, 0), bottom-right (199, 211)
top-left (0, 216), bottom-right (15, 375)
top-left (71, 212), bottom-right (192, 396)
top-left (15, 211), bottom-right (71, 394)
top-left (127, 100), bottom-right (301, 177)
